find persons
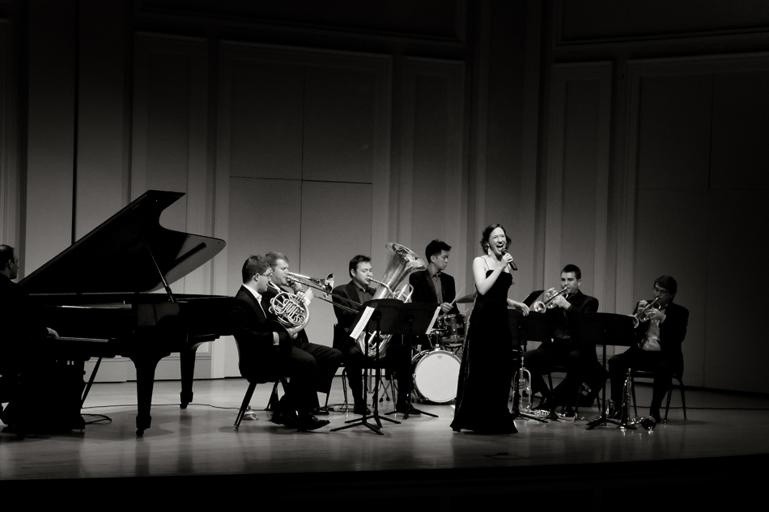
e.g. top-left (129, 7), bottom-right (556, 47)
top-left (603, 276), bottom-right (689, 431)
top-left (449, 224), bottom-right (531, 434)
top-left (261, 251), bottom-right (343, 416)
top-left (409, 239), bottom-right (465, 349)
top-left (0, 244), bottom-right (60, 440)
top-left (331, 255), bottom-right (422, 415)
top-left (522, 264), bottom-right (599, 417)
top-left (233, 255), bottom-right (343, 431)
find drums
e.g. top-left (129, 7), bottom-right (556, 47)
top-left (438, 313), bottom-right (466, 347)
top-left (412, 350), bottom-right (460, 403)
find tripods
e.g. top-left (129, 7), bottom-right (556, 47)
top-left (344, 321), bottom-right (401, 428)
top-left (384, 331), bottom-right (439, 420)
top-left (586, 345), bottom-right (638, 430)
top-left (510, 345), bottom-right (548, 425)
top-left (330, 321), bottom-right (384, 435)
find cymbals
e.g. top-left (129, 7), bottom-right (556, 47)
top-left (456, 294), bottom-right (476, 303)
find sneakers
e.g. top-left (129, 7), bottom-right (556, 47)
top-left (353, 405), bottom-right (370, 415)
top-left (271, 409), bottom-right (297, 426)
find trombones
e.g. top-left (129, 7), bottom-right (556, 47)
top-left (284, 273), bottom-right (361, 314)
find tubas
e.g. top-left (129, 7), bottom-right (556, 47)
top-left (358, 242), bottom-right (426, 357)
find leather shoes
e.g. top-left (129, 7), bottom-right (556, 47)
top-left (312, 406), bottom-right (329, 415)
top-left (297, 415), bottom-right (329, 429)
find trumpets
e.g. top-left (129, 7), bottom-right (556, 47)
top-left (628, 296), bottom-right (661, 327)
top-left (639, 416), bottom-right (656, 431)
top-left (623, 368), bottom-right (637, 426)
top-left (534, 288), bottom-right (570, 312)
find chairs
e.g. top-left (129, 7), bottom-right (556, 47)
top-left (233, 374), bottom-right (289, 433)
top-left (325, 324), bottom-right (397, 413)
top-left (547, 368), bottom-right (601, 413)
top-left (631, 371), bottom-right (686, 425)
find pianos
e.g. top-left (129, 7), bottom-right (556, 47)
top-left (14, 189), bottom-right (236, 440)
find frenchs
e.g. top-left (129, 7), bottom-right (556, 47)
top-left (267, 280), bottom-right (309, 335)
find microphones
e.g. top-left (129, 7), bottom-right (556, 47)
top-left (501, 248), bottom-right (517, 271)
